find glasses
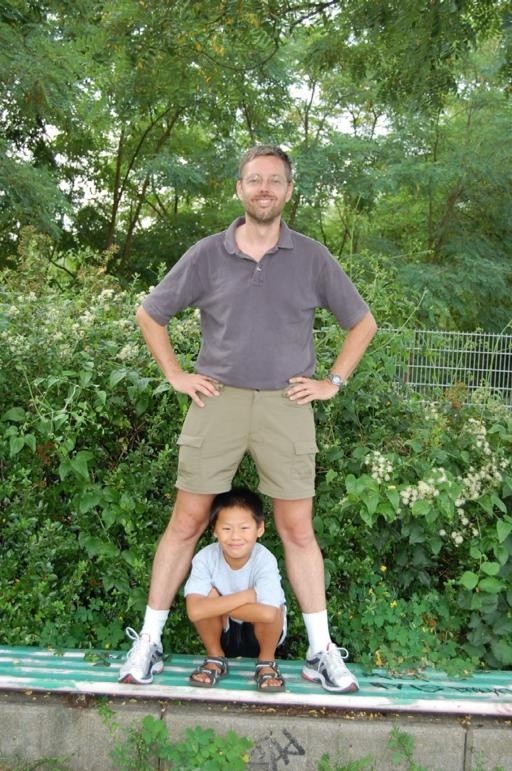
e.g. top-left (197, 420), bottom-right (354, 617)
top-left (247, 174), bottom-right (282, 187)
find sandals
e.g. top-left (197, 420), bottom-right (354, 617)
top-left (254, 660), bottom-right (285, 692)
top-left (187, 655), bottom-right (229, 687)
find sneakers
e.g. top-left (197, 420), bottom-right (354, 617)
top-left (119, 633), bottom-right (164, 684)
top-left (300, 643), bottom-right (359, 693)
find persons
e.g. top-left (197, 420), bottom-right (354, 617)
top-left (184, 486), bottom-right (288, 692)
top-left (120, 144), bottom-right (379, 693)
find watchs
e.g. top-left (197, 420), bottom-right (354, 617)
top-left (326, 373), bottom-right (344, 386)
